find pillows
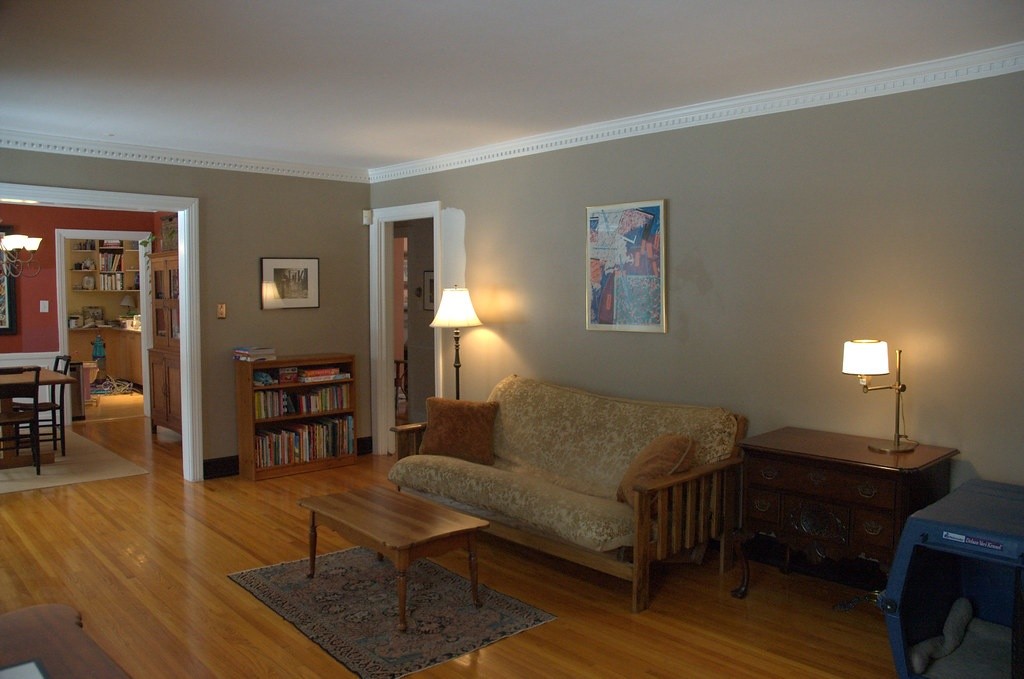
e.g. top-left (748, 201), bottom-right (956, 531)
top-left (614, 429), bottom-right (698, 513)
top-left (417, 395), bottom-right (500, 467)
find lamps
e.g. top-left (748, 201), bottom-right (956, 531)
top-left (835, 334), bottom-right (921, 456)
top-left (0, 233), bottom-right (44, 279)
top-left (120, 294), bottom-right (138, 317)
top-left (427, 284), bottom-right (484, 401)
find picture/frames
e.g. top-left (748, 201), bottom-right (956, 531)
top-left (0, 223), bottom-right (18, 338)
top-left (259, 256), bottom-right (322, 311)
top-left (422, 269), bottom-right (435, 312)
top-left (580, 196), bottom-right (676, 339)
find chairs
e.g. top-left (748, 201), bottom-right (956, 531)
top-left (35, 354), bottom-right (72, 458)
top-left (0, 366), bottom-right (42, 476)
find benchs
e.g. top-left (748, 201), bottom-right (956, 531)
top-left (386, 372), bottom-right (749, 614)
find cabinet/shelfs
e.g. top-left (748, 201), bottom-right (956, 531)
top-left (237, 349), bottom-right (360, 483)
top-left (732, 425), bottom-right (961, 601)
top-left (147, 348), bottom-right (181, 437)
top-left (70, 329), bottom-right (146, 390)
top-left (147, 251), bottom-right (181, 351)
top-left (69, 236), bottom-right (141, 293)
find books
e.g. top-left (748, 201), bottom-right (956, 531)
top-left (254, 389), bottom-right (287, 419)
top-left (255, 415), bottom-right (354, 469)
top-left (297, 368), bottom-right (350, 383)
top-left (232, 346), bottom-right (276, 362)
top-left (297, 383), bottom-right (350, 414)
top-left (100, 274), bottom-right (122, 290)
top-left (99, 253), bottom-right (121, 271)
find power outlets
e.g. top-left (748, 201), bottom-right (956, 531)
top-left (216, 303), bottom-right (226, 319)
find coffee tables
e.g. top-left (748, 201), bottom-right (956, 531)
top-left (292, 481), bottom-right (490, 634)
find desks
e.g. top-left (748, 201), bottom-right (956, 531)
top-left (0, 601), bottom-right (128, 679)
top-left (0, 361), bottom-right (77, 471)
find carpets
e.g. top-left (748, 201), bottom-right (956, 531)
top-left (225, 545), bottom-right (559, 679)
top-left (0, 422), bottom-right (150, 495)
